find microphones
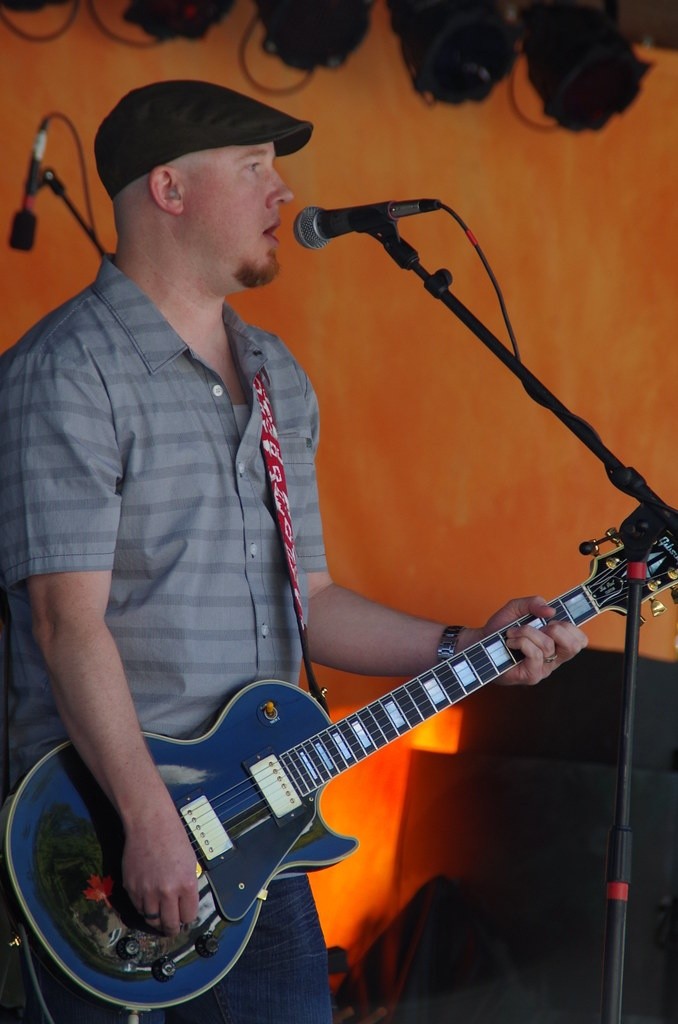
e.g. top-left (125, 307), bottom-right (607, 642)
top-left (10, 117), bottom-right (51, 250)
top-left (292, 197), bottom-right (443, 250)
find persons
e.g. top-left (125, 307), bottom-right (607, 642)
top-left (1, 78), bottom-right (589, 1024)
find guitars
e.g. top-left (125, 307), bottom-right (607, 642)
top-left (0, 527), bottom-right (678, 1015)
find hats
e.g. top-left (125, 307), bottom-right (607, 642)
top-left (94, 78), bottom-right (313, 199)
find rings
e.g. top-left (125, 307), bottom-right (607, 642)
top-left (544, 652), bottom-right (558, 663)
top-left (144, 912), bottom-right (160, 919)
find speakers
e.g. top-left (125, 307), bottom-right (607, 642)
top-left (373, 649), bottom-right (678, 1024)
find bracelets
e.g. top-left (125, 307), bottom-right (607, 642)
top-left (437, 626), bottom-right (469, 664)
